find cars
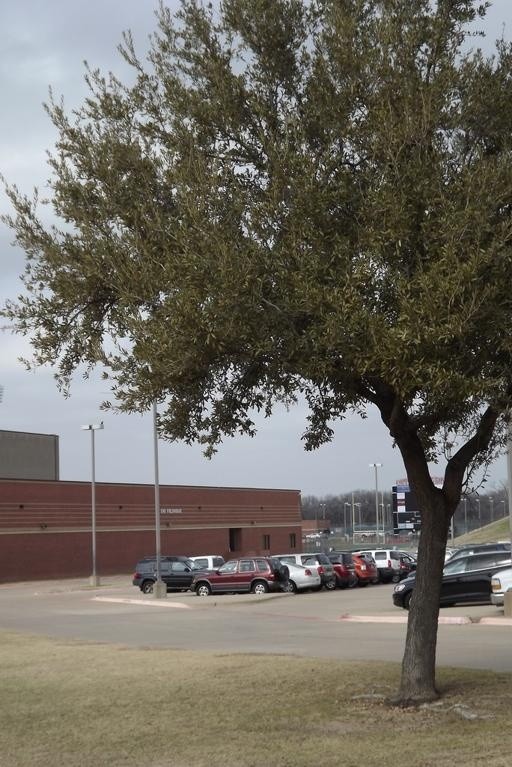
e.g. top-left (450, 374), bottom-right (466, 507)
top-left (305, 532), bottom-right (322, 539)
top-left (271, 541), bottom-right (512, 611)
top-left (189, 555), bottom-right (226, 571)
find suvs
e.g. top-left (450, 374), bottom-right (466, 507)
top-left (190, 556), bottom-right (290, 597)
top-left (133, 554), bottom-right (208, 593)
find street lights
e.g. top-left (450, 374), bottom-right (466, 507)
top-left (319, 502), bottom-right (326, 520)
top-left (343, 461), bottom-right (506, 549)
top-left (80, 422), bottom-right (105, 587)
